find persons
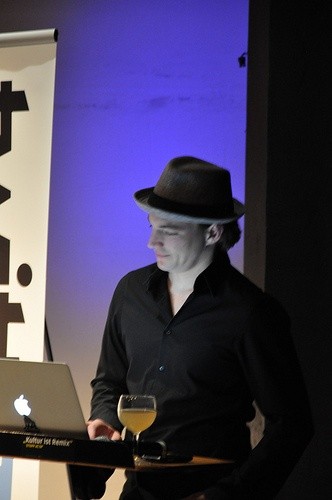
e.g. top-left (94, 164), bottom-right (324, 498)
top-left (85, 156), bottom-right (315, 500)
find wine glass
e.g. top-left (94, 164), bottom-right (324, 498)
top-left (116, 394), bottom-right (156, 465)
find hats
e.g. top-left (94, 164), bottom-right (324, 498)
top-left (132, 157), bottom-right (246, 223)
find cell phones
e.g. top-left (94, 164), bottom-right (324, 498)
top-left (142, 454), bottom-right (194, 464)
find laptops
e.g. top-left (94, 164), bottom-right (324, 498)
top-left (0, 357), bottom-right (91, 441)
